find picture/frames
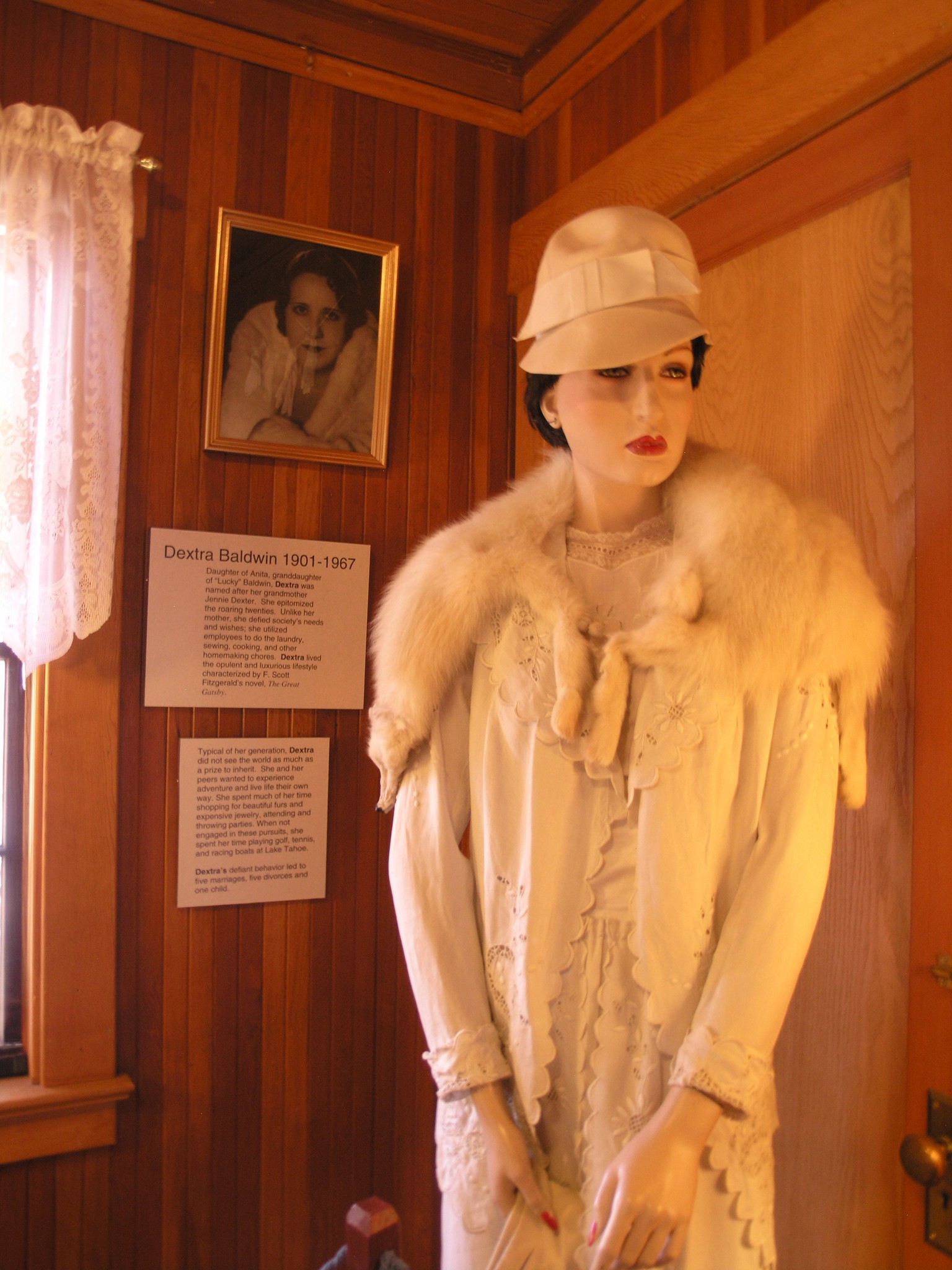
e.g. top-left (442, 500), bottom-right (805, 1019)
top-left (200, 202), bottom-right (402, 469)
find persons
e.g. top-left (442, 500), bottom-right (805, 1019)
top-left (361, 203), bottom-right (895, 1270)
top-left (221, 246), bottom-right (376, 453)
